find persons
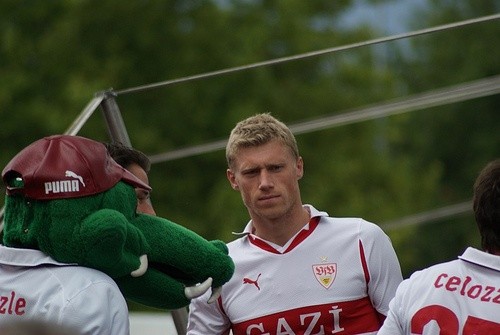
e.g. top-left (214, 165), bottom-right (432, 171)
top-left (0, 135), bottom-right (152, 335)
top-left (377, 158), bottom-right (500, 334)
top-left (184, 112), bottom-right (405, 335)
top-left (98, 143), bottom-right (186, 335)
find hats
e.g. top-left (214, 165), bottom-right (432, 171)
top-left (2, 132), bottom-right (153, 200)
